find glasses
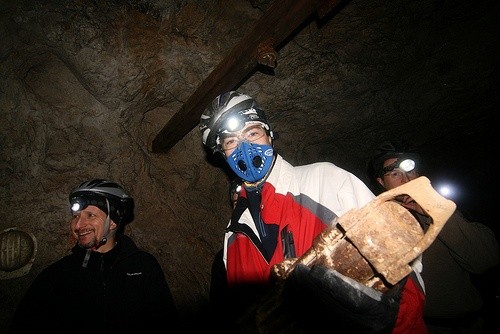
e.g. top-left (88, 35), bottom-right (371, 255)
top-left (221, 125), bottom-right (266, 151)
top-left (385, 169), bottom-right (417, 179)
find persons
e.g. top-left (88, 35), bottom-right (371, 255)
top-left (7, 179), bottom-right (180, 334)
top-left (199, 89), bottom-right (431, 334)
top-left (376, 148), bottom-right (500, 334)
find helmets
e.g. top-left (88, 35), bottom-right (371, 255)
top-left (69, 178), bottom-right (135, 227)
top-left (199, 91), bottom-right (273, 162)
